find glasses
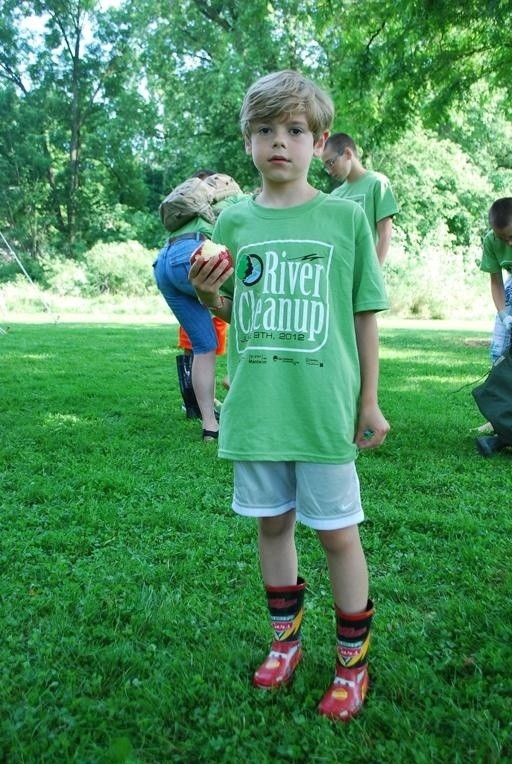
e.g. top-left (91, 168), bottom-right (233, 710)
top-left (325, 152), bottom-right (338, 169)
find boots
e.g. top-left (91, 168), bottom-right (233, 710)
top-left (317, 598), bottom-right (373, 720)
top-left (253, 576), bottom-right (305, 689)
top-left (176, 355), bottom-right (201, 419)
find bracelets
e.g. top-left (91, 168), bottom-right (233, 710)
top-left (200, 295), bottom-right (225, 311)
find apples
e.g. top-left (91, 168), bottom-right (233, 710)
top-left (190, 239), bottom-right (232, 279)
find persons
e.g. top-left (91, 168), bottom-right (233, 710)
top-left (479, 197), bottom-right (512, 367)
top-left (153, 170), bottom-right (262, 442)
top-left (320, 133), bottom-right (399, 267)
top-left (187, 69), bottom-right (391, 721)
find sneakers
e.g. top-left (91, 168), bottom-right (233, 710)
top-left (476, 437), bottom-right (500, 456)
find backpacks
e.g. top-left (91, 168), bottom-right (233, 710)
top-left (159, 172), bottom-right (242, 233)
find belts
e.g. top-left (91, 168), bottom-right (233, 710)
top-left (169, 233), bottom-right (205, 245)
